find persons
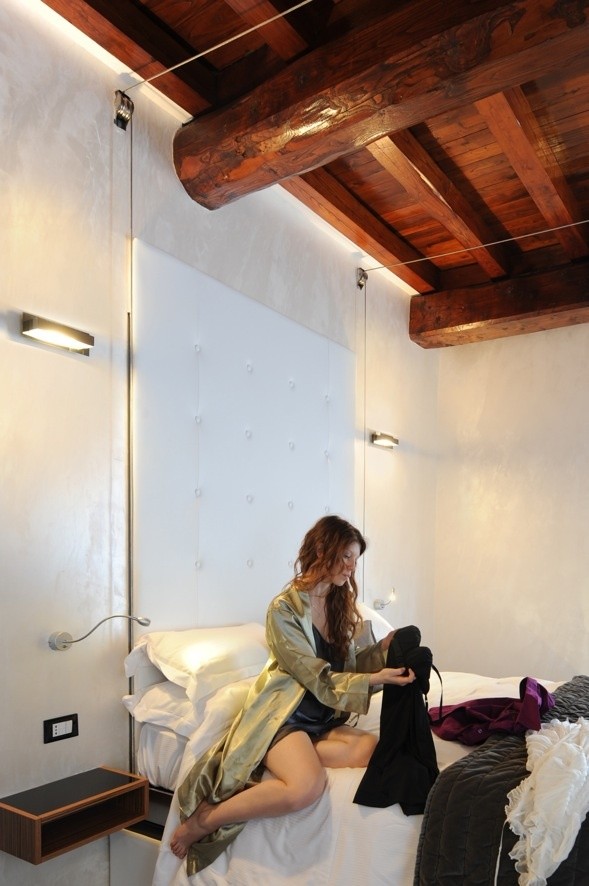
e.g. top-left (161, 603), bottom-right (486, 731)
top-left (171, 515), bottom-right (416, 858)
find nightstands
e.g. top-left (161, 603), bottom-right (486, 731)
top-left (0, 767), bottom-right (151, 865)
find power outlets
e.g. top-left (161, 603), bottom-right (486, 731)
top-left (44, 713), bottom-right (79, 744)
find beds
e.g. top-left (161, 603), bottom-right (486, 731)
top-left (134, 672), bottom-right (589, 886)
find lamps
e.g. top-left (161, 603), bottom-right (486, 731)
top-left (21, 311), bottom-right (95, 357)
top-left (372, 432), bottom-right (399, 449)
top-left (48, 614), bottom-right (151, 651)
top-left (374, 588), bottom-right (396, 609)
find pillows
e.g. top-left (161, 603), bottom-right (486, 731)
top-left (124, 622), bottom-right (278, 702)
top-left (122, 675), bottom-right (259, 742)
